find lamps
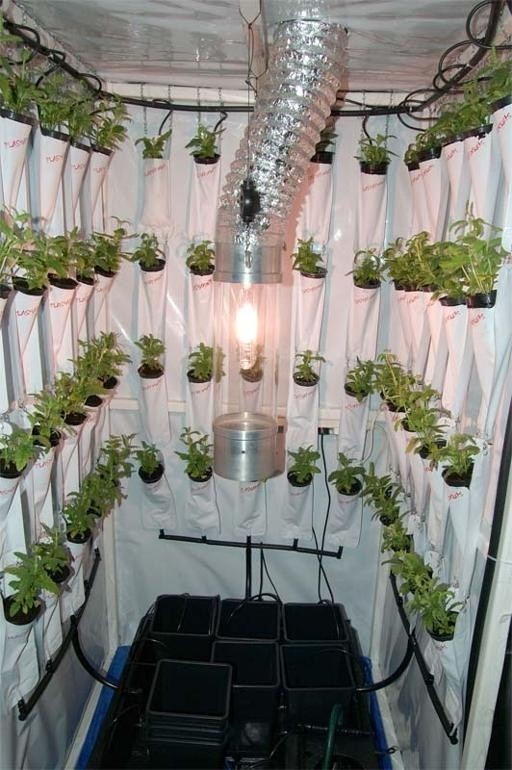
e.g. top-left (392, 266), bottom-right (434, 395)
top-left (234, 303), bottom-right (266, 383)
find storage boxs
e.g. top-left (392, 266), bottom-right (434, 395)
top-left (231, 676), bottom-right (277, 727)
top-left (282, 688), bottom-right (382, 733)
top-left (284, 735), bottom-right (389, 767)
top-left (137, 664), bottom-right (236, 764)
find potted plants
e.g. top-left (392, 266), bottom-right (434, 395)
top-left (0, 1), bottom-right (512, 669)
